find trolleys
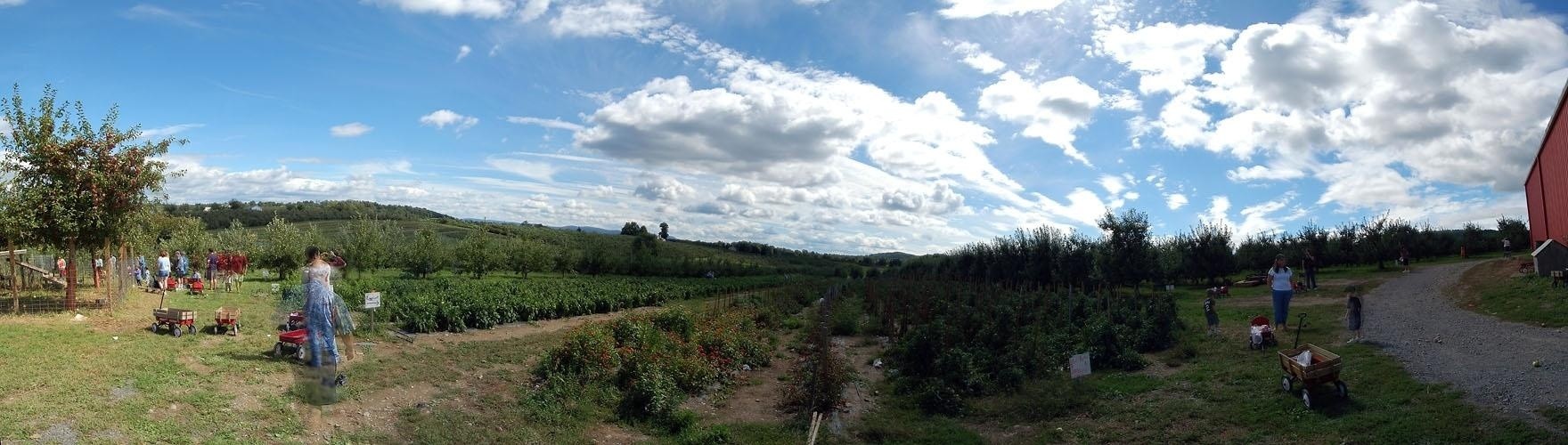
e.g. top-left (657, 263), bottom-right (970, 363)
top-left (213, 305), bottom-right (242, 337)
top-left (1249, 313), bottom-right (1276, 351)
top-left (1277, 312), bottom-right (1349, 409)
top-left (151, 288), bottom-right (198, 338)
top-left (273, 311), bottom-right (311, 361)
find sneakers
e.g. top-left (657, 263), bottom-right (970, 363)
top-left (1205, 326), bottom-right (1220, 335)
top-left (1344, 334), bottom-right (1365, 344)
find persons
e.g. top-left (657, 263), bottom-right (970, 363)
top-left (301, 246), bottom-right (356, 368)
top-left (1501, 237), bottom-right (1511, 259)
top-left (110, 253), bottom-right (116, 265)
top-left (1267, 254), bottom-right (1297, 333)
top-left (96, 254), bottom-right (103, 268)
top-left (1301, 248), bottom-right (1319, 290)
top-left (1203, 289), bottom-right (1220, 336)
top-left (1461, 245), bottom-right (1466, 260)
top-left (1344, 285), bottom-right (1363, 344)
top-left (133, 247), bottom-right (248, 296)
top-left (56, 256), bottom-right (67, 278)
top-left (1402, 247), bottom-right (1410, 273)
